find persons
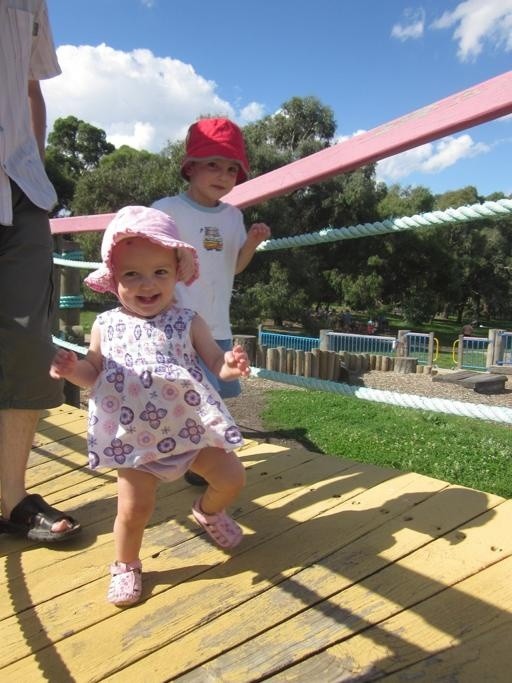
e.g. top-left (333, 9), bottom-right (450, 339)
top-left (149, 118), bottom-right (272, 484)
top-left (462, 320), bottom-right (473, 336)
top-left (312, 302), bottom-right (380, 334)
top-left (1, 0), bottom-right (84, 546)
top-left (50, 203), bottom-right (251, 606)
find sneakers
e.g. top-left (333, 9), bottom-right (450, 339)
top-left (192, 495), bottom-right (243, 549)
top-left (184, 470), bottom-right (207, 486)
top-left (107, 559), bottom-right (143, 605)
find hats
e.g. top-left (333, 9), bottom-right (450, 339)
top-left (84, 206), bottom-right (198, 293)
top-left (180, 118), bottom-right (249, 186)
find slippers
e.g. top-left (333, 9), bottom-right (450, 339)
top-left (0, 494), bottom-right (82, 544)
